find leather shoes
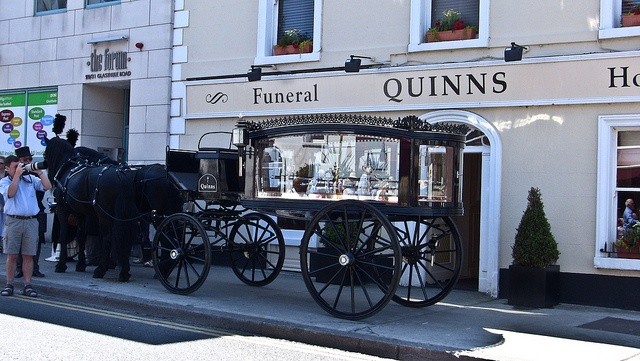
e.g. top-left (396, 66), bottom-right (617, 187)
top-left (13, 269), bottom-right (24, 278)
top-left (33, 270), bottom-right (45, 277)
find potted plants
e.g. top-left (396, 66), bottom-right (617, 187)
top-left (621, 5), bottom-right (640, 26)
top-left (507, 186), bottom-right (561, 307)
top-left (320, 219), bottom-right (361, 284)
top-left (614, 222), bottom-right (640, 259)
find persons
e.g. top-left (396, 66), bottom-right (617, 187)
top-left (14, 144), bottom-right (48, 278)
top-left (0, 154), bottom-right (48, 297)
top-left (0, 157), bottom-right (8, 256)
top-left (622, 197), bottom-right (638, 233)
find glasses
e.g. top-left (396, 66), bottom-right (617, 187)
top-left (23, 158), bottom-right (34, 162)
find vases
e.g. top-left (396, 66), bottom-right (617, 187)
top-left (274, 42), bottom-right (312, 55)
top-left (426, 28), bottom-right (475, 42)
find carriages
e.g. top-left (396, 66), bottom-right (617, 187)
top-left (42, 116), bottom-right (470, 322)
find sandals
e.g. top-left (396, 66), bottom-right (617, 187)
top-left (21, 284), bottom-right (38, 296)
top-left (1, 284), bottom-right (13, 296)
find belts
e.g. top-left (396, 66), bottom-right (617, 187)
top-left (6, 215), bottom-right (37, 220)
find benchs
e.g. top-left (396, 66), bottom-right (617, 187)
top-left (195, 132), bottom-right (239, 200)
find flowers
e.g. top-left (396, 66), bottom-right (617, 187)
top-left (275, 28), bottom-right (311, 48)
top-left (428, 9), bottom-right (468, 41)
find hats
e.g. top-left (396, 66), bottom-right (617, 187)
top-left (13, 146), bottom-right (35, 158)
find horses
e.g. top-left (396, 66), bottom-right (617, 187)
top-left (40, 132), bottom-right (183, 284)
top-left (73, 144), bottom-right (195, 274)
